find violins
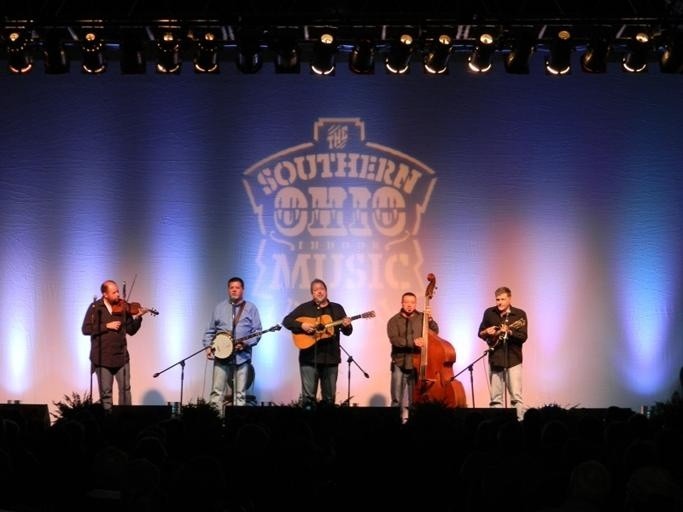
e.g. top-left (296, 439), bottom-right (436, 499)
top-left (111, 299), bottom-right (158, 317)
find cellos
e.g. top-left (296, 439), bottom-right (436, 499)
top-left (413, 273), bottom-right (467, 408)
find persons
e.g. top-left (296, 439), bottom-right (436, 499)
top-left (476, 285), bottom-right (525, 419)
top-left (0, 403), bottom-right (682, 508)
top-left (281, 279), bottom-right (353, 410)
top-left (81, 280), bottom-right (151, 404)
top-left (385, 292), bottom-right (439, 406)
top-left (199, 275), bottom-right (263, 409)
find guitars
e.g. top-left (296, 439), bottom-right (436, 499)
top-left (486, 318), bottom-right (525, 348)
top-left (293, 310), bottom-right (376, 349)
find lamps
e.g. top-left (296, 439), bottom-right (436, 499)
top-left (1, 22), bottom-right (682, 78)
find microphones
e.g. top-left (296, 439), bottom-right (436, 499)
top-left (230, 296), bottom-right (236, 304)
top-left (210, 340), bottom-right (216, 347)
top-left (92, 296), bottom-right (96, 312)
top-left (316, 296), bottom-right (322, 310)
top-left (413, 308), bottom-right (421, 315)
top-left (122, 280), bottom-right (125, 298)
top-left (505, 310), bottom-right (511, 323)
top-left (483, 347), bottom-right (495, 352)
